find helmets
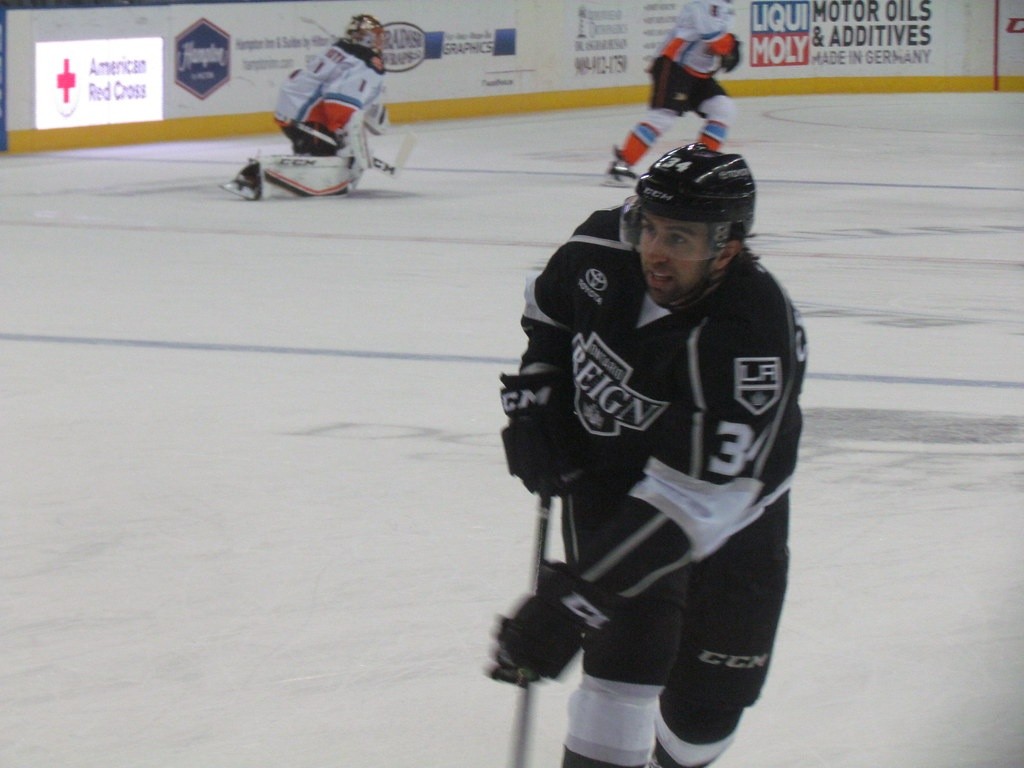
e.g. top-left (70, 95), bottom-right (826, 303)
top-left (346, 16), bottom-right (382, 52)
top-left (635, 142), bottom-right (756, 243)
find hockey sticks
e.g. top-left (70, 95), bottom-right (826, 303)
top-left (507, 495), bottom-right (553, 767)
top-left (370, 132), bottom-right (418, 180)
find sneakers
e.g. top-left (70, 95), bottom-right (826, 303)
top-left (602, 159), bottom-right (638, 188)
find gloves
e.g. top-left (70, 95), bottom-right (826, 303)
top-left (490, 570), bottom-right (628, 688)
top-left (722, 40), bottom-right (740, 73)
top-left (497, 367), bottom-right (598, 497)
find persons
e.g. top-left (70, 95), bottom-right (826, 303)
top-left (486, 142), bottom-right (808, 768)
top-left (601, 0), bottom-right (741, 188)
top-left (221, 13), bottom-right (389, 200)
